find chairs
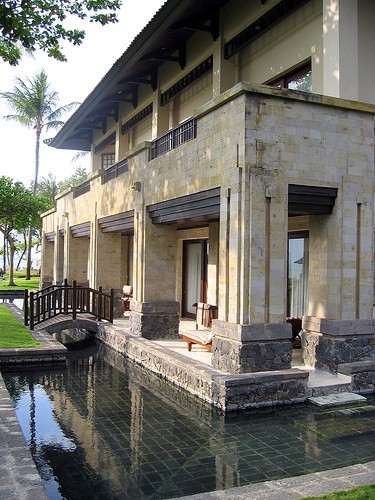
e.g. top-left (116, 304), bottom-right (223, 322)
top-left (181, 330), bottom-right (214, 352)
top-left (286, 316), bottom-right (303, 342)
top-left (122, 285), bottom-right (133, 311)
